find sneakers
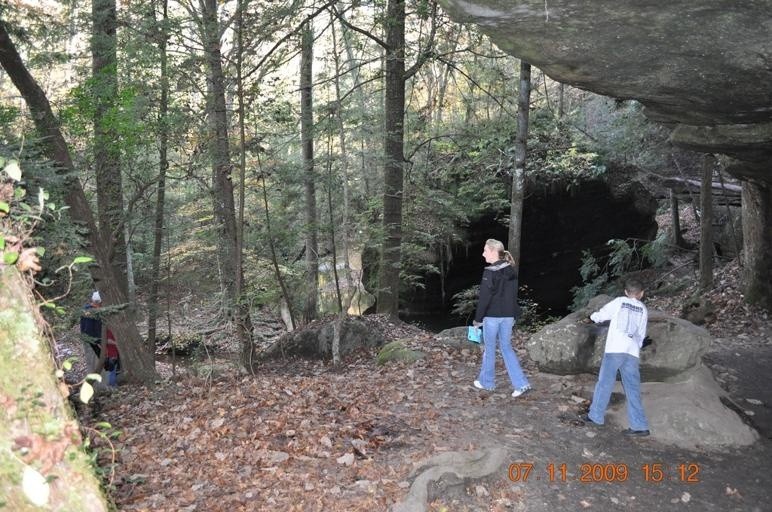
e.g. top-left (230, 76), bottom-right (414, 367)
top-left (474, 381), bottom-right (483, 389)
top-left (511, 385), bottom-right (531, 397)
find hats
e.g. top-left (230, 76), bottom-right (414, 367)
top-left (92, 292), bottom-right (101, 304)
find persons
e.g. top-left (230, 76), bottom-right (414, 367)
top-left (78, 293), bottom-right (120, 386)
top-left (583, 278), bottom-right (651, 437)
top-left (473, 238), bottom-right (531, 399)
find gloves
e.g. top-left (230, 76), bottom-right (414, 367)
top-left (642, 336), bottom-right (652, 348)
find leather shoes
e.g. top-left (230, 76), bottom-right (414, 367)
top-left (622, 428), bottom-right (649, 436)
